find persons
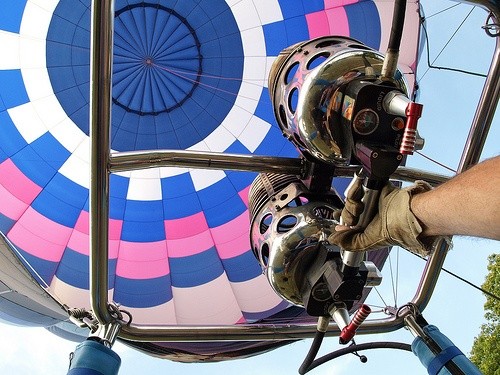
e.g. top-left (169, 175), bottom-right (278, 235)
top-left (326, 153), bottom-right (500, 257)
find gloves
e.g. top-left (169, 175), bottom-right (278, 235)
top-left (328, 174), bottom-right (453, 257)
